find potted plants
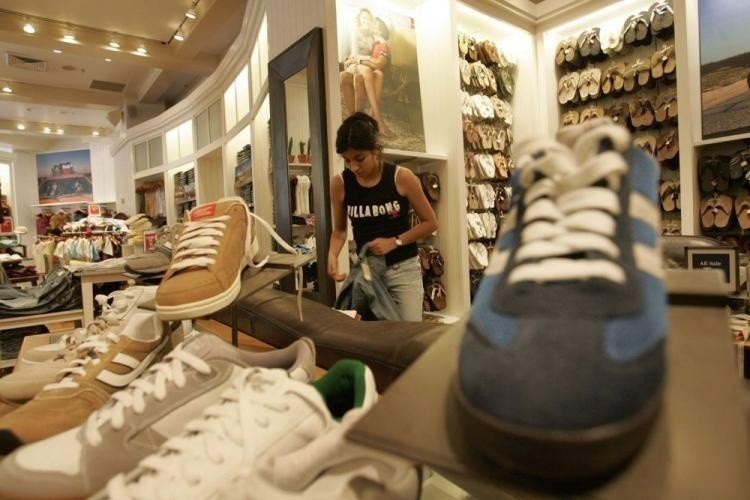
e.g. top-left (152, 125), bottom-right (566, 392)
top-left (288, 138), bottom-right (294, 162)
top-left (307, 138), bottom-right (310, 163)
top-left (298, 142), bottom-right (308, 162)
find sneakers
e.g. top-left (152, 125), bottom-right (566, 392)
top-left (86, 358), bottom-right (421, 500)
top-left (20, 286), bottom-right (160, 368)
top-left (155, 196), bottom-right (304, 321)
top-left (0, 319), bottom-right (174, 457)
top-left (124, 224), bottom-right (186, 275)
top-left (0, 313), bottom-right (136, 410)
top-left (448, 116), bottom-right (667, 488)
top-left (1, 329), bottom-right (316, 499)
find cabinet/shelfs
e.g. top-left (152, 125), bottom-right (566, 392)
top-left (0, 195), bottom-right (41, 288)
top-left (0, 259), bottom-right (135, 371)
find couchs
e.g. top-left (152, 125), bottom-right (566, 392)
top-left (210, 284), bottom-right (450, 392)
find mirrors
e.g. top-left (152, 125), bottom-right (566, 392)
top-left (267, 25), bottom-right (336, 310)
top-left (1, 246), bottom-right (750, 498)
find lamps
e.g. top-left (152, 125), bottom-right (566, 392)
top-left (175, 30), bottom-right (184, 41)
top-left (184, 8), bottom-right (197, 20)
top-left (1, 80), bottom-right (14, 93)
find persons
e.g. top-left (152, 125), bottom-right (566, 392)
top-left (326, 112), bottom-right (439, 321)
top-left (344, 7), bottom-right (396, 139)
top-left (339, 16), bottom-right (391, 117)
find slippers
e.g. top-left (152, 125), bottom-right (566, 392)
top-left (578, 31), bottom-right (589, 56)
top-left (575, 59), bottom-right (587, 68)
top-left (567, 71), bottom-right (579, 100)
top-left (430, 253), bottom-right (442, 275)
top-left (580, 109), bottom-right (590, 123)
top-left (676, 183), bottom-right (682, 211)
top-left (598, 54), bottom-right (607, 61)
top-left (648, 3), bottom-right (662, 31)
top-left (459, 88), bottom-right (512, 124)
top-left (630, 96), bottom-right (642, 127)
top-left (464, 148), bottom-right (511, 183)
top-left (568, 111), bottom-right (578, 126)
top-left (436, 255), bottom-right (444, 274)
top-left (613, 63), bottom-right (625, 91)
top-left (590, 27), bottom-right (600, 55)
top-left (426, 281), bottom-right (440, 310)
top-left (558, 74), bottom-right (569, 105)
top-left (601, 19), bottom-right (611, 55)
top-left (729, 153), bottom-right (743, 189)
top-left (701, 195), bottom-right (714, 230)
top-left (459, 58), bottom-right (513, 99)
top-left (637, 58), bottom-right (650, 85)
top-left (419, 248), bottom-right (429, 269)
top-left (655, 94), bottom-right (667, 122)
top-left (620, 45), bottom-right (630, 56)
top-left (602, 66), bottom-right (612, 94)
top-left (715, 155), bottom-right (728, 191)
top-left (579, 70), bottom-right (589, 100)
top-left (642, 93), bottom-right (655, 126)
top-left (464, 119), bottom-right (515, 153)
top-left (465, 180), bottom-right (514, 211)
top-left (617, 103), bottom-right (629, 125)
top-left (560, 113), bottom-right (568, 127)
top-left (664, 45), bottom-right (676, 73)
top-left (612, 17), bottom-right (622, 52)
top-left (662, 0), bottom-right (674, 29)
top-left (555, 40), bottom-right (565, 64)
top-left (624, 62), bottom-right (635, 91)
top-left (467, 241), bottom-right (497, 272)
top-left (735, 193), bottom-right (750, 229)
top-left (660, 180), bottom-right (674, 212)
top-left (741, 150), bottom-right (750, 189)
top-left (668, 95), bottom-right (678, 118)
top-left (590, 106), bottom-right (604, 120)
top-left (641, 135), bottom-right (656, 155)
top-left (665, 132), bottom-right (679, 159)
top-left (657, 132), bottom-right (666, 160)
top-left (715, 193), bottom-right (732, 229)
top-left (458, 34), bottom-right (516, 72)
top-left (565, 36), bottom-right (578, 62)
top-left (421, 288), bottom-right (431, 312)
top-left (421, 173), bottom-right (438, 200)
top-left (590, 68), bottom-right (601, 95)
top-left (667, 74), bottom-right (676, 81)
top-left (636, 11), bottom-right (649, 41)
top-left (652, 51), bottom-right (663, 78)
top-left (436, 282), bottom-right (447, 309)
top-left (699, 156), bottom-right (715, 191)
top-left (623, 14), bottom-right (636, 44)
top-left (431, 173), bottom-right (440, 202)
top-left (466, 211), bottom-right (507, 240)
top-left (609, 105), bottom-right (617, 125)
top-left (633, 137), bottom-right (642, 151)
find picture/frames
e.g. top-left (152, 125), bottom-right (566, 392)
top-left (683, 244), bottom-right (742, 297)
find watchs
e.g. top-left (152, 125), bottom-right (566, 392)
top-left (394, 235), bottom-right (404, 250)
